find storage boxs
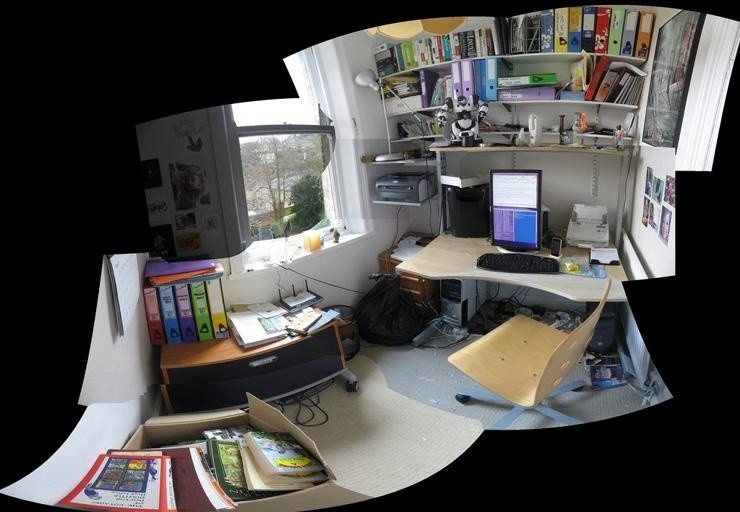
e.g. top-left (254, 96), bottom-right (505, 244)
top-left (118, 390), bottom-right (373, 511)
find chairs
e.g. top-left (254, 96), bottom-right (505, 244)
top-left (446, 279), bottom-right (612, 430)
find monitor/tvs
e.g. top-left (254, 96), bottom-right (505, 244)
top-left (490, 168), bottom-right (542, 252)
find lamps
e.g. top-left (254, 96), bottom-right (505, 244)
top-left (354, 67), bottom-right (422, 163)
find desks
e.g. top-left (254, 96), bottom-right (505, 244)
top-left (395, 228), bottom-right (629, 347)
top-left (157, 303), bottom-right (359, 415)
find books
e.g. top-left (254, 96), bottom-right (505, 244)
top-left (56, 424), bottom-right (329, 512)
top-left (614, 76), bottom-right (645, 106)
top-left (375, 27), bottom-right (495, 77)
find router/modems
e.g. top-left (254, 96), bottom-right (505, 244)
top-left (279, 279), bottom-right (324, 314)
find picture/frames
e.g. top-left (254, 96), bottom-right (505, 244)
top-left (641, 10), bottom-right (707, 150)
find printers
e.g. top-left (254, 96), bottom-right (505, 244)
top-left (375, 170), bottom-right (437, 204)
top-left (567, 208), bottom-right (610, 249)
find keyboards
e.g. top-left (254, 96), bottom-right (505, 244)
top-left (475, 252), bottom-right (560, 274)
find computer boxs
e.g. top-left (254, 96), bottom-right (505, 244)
top-left (440, 278), bottom-right (488, 327)
top-left (583, 302), bottom-right (618, 359)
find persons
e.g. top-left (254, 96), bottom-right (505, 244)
top-left (660, 207), bottom-right (672, 241)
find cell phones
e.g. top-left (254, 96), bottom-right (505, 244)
top-left (551, 238), bottom-right (562, 257)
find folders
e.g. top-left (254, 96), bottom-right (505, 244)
top-left (420, 59), bottom-right (558, 108)
top-left (143, 277), bottom-right (231, 346)
top-left (509, 6), bottom-right (656, 60)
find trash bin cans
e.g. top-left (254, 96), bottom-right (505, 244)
top-left (322, 305), bottom-right (360, 361)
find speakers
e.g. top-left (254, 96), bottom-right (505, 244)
top-left (448, 186), bottom-right (490, 238)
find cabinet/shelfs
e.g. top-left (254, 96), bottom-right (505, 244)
top-left (377, 53), bottom-right (647, 144)
top-left (370, 154), bottom-right (435, 208)
top-left (376, 236), bottom-right (441, 327)
top-left (430, 140), bottom-right (632, 252)
